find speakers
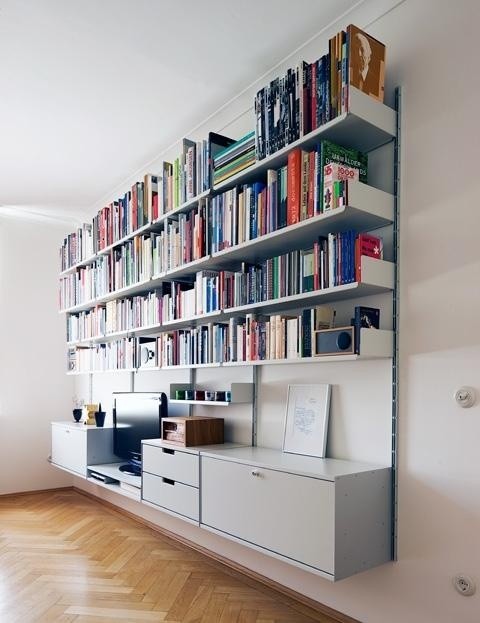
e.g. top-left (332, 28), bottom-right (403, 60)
top-left (313, 325), bottom-right (355, 356)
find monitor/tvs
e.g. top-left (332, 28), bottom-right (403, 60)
top-left (113, 392), bottom-right (168, 475)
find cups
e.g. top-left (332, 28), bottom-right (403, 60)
top-left (174, 390), bottom-right (231, 402)
top-left (94, 412), bottom-right (106, 427)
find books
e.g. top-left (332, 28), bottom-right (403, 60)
top-left (59, 23), bottom-right (385, 371)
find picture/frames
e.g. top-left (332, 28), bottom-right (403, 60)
top-left (281, 382), bottom-right (332, 458)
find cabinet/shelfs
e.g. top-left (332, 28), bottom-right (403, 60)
top-left (140, 438), bottom-right (202, 524)
top-left (49, 419), bottom-right (115, 478)
top-left (200, 446), bottom-right (394, 585)
top-left (59, 85), bottom-right (398, 377)
